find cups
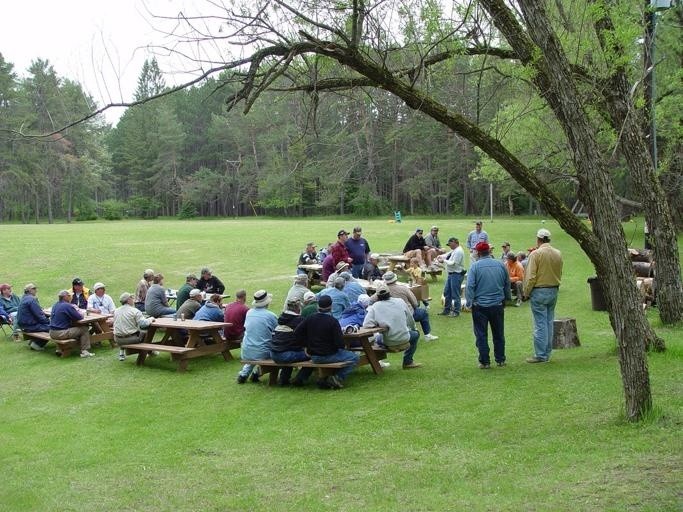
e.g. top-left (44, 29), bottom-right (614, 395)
top-left (181, 313), bottom-right (185, 320)
top-left (173, 314), bottom-right (178, 320)
top-left (86, 309), bottom-right (91, 315)
top-left (99, 307), bottom-right (104, 314)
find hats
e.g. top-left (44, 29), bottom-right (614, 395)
top-left (187, 267), bottom-right (212, 300)
top-left (416, 221), bottom-right (551, 259)
top-left (0, 278), bottom-right (134, 304)
top-left (252, 227), bottom-right (398, 315)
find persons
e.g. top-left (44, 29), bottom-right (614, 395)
top-left (523, 228), bottom-right (564, 364)
top-left (325, 260), bottom-right (349, 287)
top-left (49, 290), bottom-right (95, 358)
top-left (133, 269), bottom-right (154, 312)
top-left (299, 291), bottom-right (320, 317)
top-left (345, 227), bottom-right (371, 278)
top-left (423, 227), bottom-right (447, 272)
top-left (144, 274), bottom-right (175, 318)
top-left (487, 244), bottom-right (495, 260)
top-left (363, 253), bottom-right (383, 281)
top-left (400, 257), bottom-right (430, 311)
top-left (17, 282), bottom-right (50, 351)
top-left (269, 299), bottom-right (314, 386)
top-left (197, 268), bottom-right (225, 295)
top-left (192, 294), bottom-right (225, 336)
top-left (498, 241), bottom-right (513, 264)
top-left (526, 246), bottom-right (537, 258)
top-left (0, 283), bottom-right (21, 342)
top-left (436, 236), bottom-right (467, 318)
top-left (337, 294), bottom-right (370, 332)
top-left (112, 291), bottom-right (156, 361)
top-left (297, 242), bottom-right (322, 288)
top-left (501, 252), bottom-right (525, 307)
top-left (283, 273), bottom-right (316, 313)
top-left (314, 276), bottom-right (352, 321)
top-left (361, 255), bottom-right (371, 281)
top-left (402, 228), bottom-right (439, 272)
top-left (64, 276), bottom-right (91, 310)
top-left (363, 284), bottom-right (423, 369)
top-left (331, 230), bottom-right (354, 272)
top-left (317, 247), bottom-right (326, 258)
top-left (464, 240), bottom-right (512, 370)
top-left (338, 271), bottom-right (367, 303)
top-left (175, 288), bottom-right (202, 318)
top-left (86, 281), bottom-right (116, 346)
top-left (292, 294), bottom-right (360, 389)
top-left (223, 289), bottom-right (252, 340)
top-left (320, 242), bottom-right (335, 264)
top-left (236, 289), bottom-right (279, 384)
top-left (465, 221), bottom-right (489, 267)
top-left (368, 270), bottom-right (439, 341)
top-left (516, 252), bottom-right (529, 271)
top-left (176, 273), bottom-right (198, 310)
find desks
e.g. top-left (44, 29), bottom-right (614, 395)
top-left (163, 289), bottom-right (230, 307)
top-left (299, 264), bottom-right (324, 290)
top-left (43, 306), bottom-right (116, 348)
top-left (387, 256), bottom-right (411, 280)
top-left (134, 317), bottom-right (233, 371)
top-left (268, 327), bottom-right (388, 383)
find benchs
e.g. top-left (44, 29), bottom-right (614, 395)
top-left (420, 269), bottom-right (442, 282)
top-left (16, 329), bottom-right (76, 358)
top-left (240, 358), bottom-right (354, 370)
top-left (121, 342), bottom-right (195, 355)
top-left (346, 344), bottom-right (410, 352)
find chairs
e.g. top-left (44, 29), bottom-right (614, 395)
top-left (0, 321), bottom-right (14, 337)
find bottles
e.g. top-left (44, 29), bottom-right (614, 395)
top-left (369, 276), bottom-right (373, 285)
top-left (408, 278), bottom-right (412, 287)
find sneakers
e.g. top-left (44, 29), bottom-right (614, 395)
top-left (437, 311), bottom-right (459, 317)
top-left (424, 335), bottom-right (438, 342)
top-left (514, 299), bottom-right (522, 307)
top-left (403, 363), bottom-right (420, 369)
top-left (147, 351), bottom-right (159, 357)
top-left (250, 374), bottom-right (259, 382)
top-left (238, 375), bottom-right (248, 383)
top-left (479, 353), bottom-right (549, 369)
top-left (12, 333), bottom-right (125, 361)
top-left (327, 376), bottom-right (344, 388)
top-left (379, 362), bottom-right (390, 368)
